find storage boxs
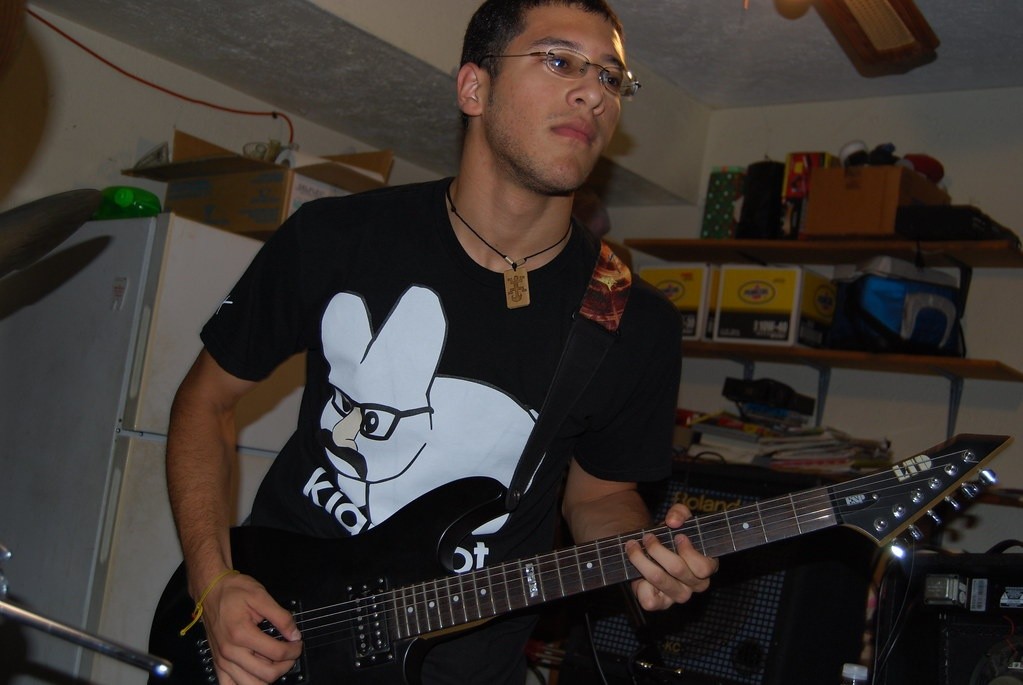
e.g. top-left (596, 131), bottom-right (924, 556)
top-left (799, 167), bottom-right (956, 238)
top-left (162, 129), bottom-right (399, 236)
top-left (632, 262), bottom-right (722, 339)
top-left (712, 262), bottom-right (835, 347)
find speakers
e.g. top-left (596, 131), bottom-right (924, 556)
top-left (557, 461), bottom-right (892, 685)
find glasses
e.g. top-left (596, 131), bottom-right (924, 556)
top-left (475, 48), bottom-right (641, 96)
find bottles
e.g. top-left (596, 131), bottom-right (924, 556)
top-left (86, 187), bottom-right (163, 225)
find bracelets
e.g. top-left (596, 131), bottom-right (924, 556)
top-left (179, 566), bottom-right (244, 636)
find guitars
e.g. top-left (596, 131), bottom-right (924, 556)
top-left (148, 432), bottom-right (1018, 685)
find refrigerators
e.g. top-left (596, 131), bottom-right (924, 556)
top-left (1, 200), bottom-right (307, 685)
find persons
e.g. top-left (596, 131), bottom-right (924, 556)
top-left (165, 1), bottom-right (722, 685)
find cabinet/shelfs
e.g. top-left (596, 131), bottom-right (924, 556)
top-left (618, 232), bottom-right (1023, 502)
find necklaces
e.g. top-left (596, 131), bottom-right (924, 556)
top-left (445, 176), bottom-right (573, 311)
